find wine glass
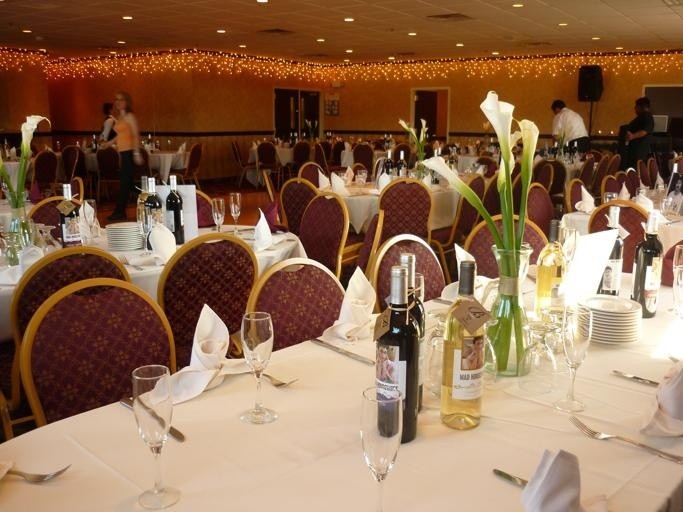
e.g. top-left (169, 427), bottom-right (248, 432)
top-left (236, 310), bottom-right (281, 427)
top-left (359, 386), bottom-right (403, 512)
top-left (128, 361), bottom-right (182, 511)
top-left (0, 218), bottom-right (59, 272)
top-left (211, 198), bottom-right (225, 234)
top-left (553, 305), bottom-right (594, 412)
top-left (82, 199), bottom-right (99, 246)
top-left (230, 193), bottom-right (242, 240)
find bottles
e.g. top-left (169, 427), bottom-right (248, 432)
top-left (138, 175), bottom-right (185, 247)
top-left (1, 132), bottom-right (161, 154)
top-left (439, 260), bottom-right (487, 432)
top-left (58, 182), bottom-right (82, 247)
top-left (374, 266), bottom-right (418, 443)
top-left (400, 252), bottom-right (425, 413)
top-left (535, 219), bottom-right (567, 324)
top-left (597, 205), bottom-right (625, 295)
top-left (630, 209), bottom-right (663, 318)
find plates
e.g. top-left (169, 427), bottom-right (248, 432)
top-left (577, 294), bottom-right (643, 346)
top-left (104, 222), bottom-right (145, 252)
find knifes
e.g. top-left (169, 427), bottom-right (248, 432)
top-left (121, 394), bottom-right (185, 442)
top-left (612, 369), bottom-right (660, 387)
top-left (308, 337), bottom-right (375, 368)
top-left (492, 469), bottom-right (528, 490)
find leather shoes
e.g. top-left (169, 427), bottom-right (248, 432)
top-left (107, 212), bottom-right (127, 221)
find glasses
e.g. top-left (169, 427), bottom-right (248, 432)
top-left (114, 98), bottom-right (126, 103)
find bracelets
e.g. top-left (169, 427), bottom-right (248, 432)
top-left (133, 152), bottom-right (140, 156)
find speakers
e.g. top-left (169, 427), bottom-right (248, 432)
top-left (577, 64), bottom-right (603, 102)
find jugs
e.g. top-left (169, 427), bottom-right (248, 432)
top-left (519, 322), bottom-right (562, 395)
top-left (426, 310), bottom-right (498, 399)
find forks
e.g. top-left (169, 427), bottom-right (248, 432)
top-left (245, 363), bottom-right (298, 390)
top-left (570, 416), bottom-right (681, 465)
top-left (7, 463), bottom-right (70, 483)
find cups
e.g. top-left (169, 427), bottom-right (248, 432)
top-left (559, 226), bottom-right (576, 263)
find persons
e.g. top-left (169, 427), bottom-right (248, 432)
top-left (551, 100), bottom-right (591, 152)
top-left (100, 91), bottom-right (145, 220)
top-left (97, 104), bottom-right (118, 142)
top-left (624, 97), bottom-right (654, 168)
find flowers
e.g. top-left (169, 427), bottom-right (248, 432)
top-left (415, 91), bottom-right (545, 376)
top-left (0, 112), bottom-right (51, 253)
top-left (396, 119), bottom-right (431, 181)
